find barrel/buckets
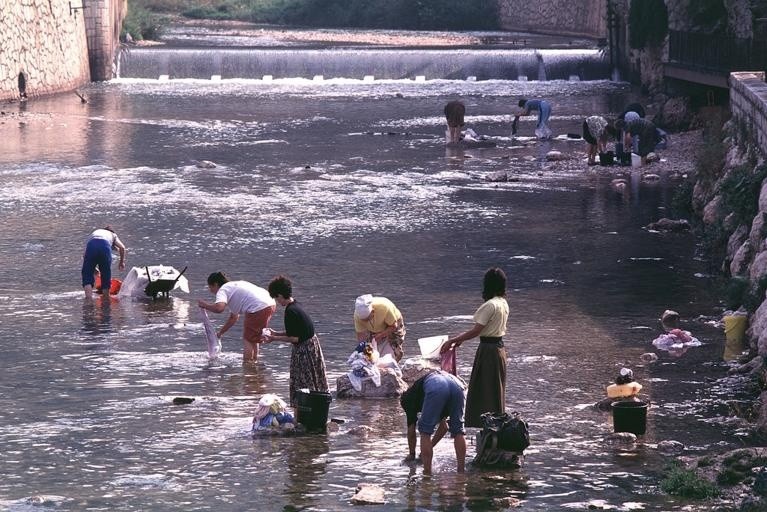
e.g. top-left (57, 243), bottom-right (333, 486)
top-left (417, 333), bottom-right (449, 359)
top-left (608, 398), bottom-right (649, 435)
top-left (295, 386), bottom-right (333, 430)
top-left (619, 151), bottom-right (632, 167)
top-left (723, 343), bottom-right (746, 362)
top-left (599, 150), bottom-right (613, 166)
top-left (721, 314), bottom-right (747, 343)
top-left (445, 130), bottom-right (451, 144)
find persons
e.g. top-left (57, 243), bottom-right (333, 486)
top-left (581, 102), bottom-right (665, 165)
top-left (351, 293), bottom-right (406, 363)
top-left (258, 275), bottom-right (331, 408)
top-left (518, 98), bottom-right (552, 141)
top-left (439, 265), bottom-right (508, 427)
top-left (197, 271), bottom-right (276, 361)
top-left (443, 100), bottom-right (465, 144)
top-left (393, 367), bottom-right (466, 474)
top-left (81, 225), bottom-right (126, 298)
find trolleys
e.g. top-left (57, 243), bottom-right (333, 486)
top-left (133, 264), bottom-right (188, 300)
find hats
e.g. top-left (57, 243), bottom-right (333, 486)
top-left (354, 293), bottom-right (374, 320)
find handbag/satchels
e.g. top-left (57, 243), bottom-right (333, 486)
top-left (475, 410), bottom-right (530, 464)
